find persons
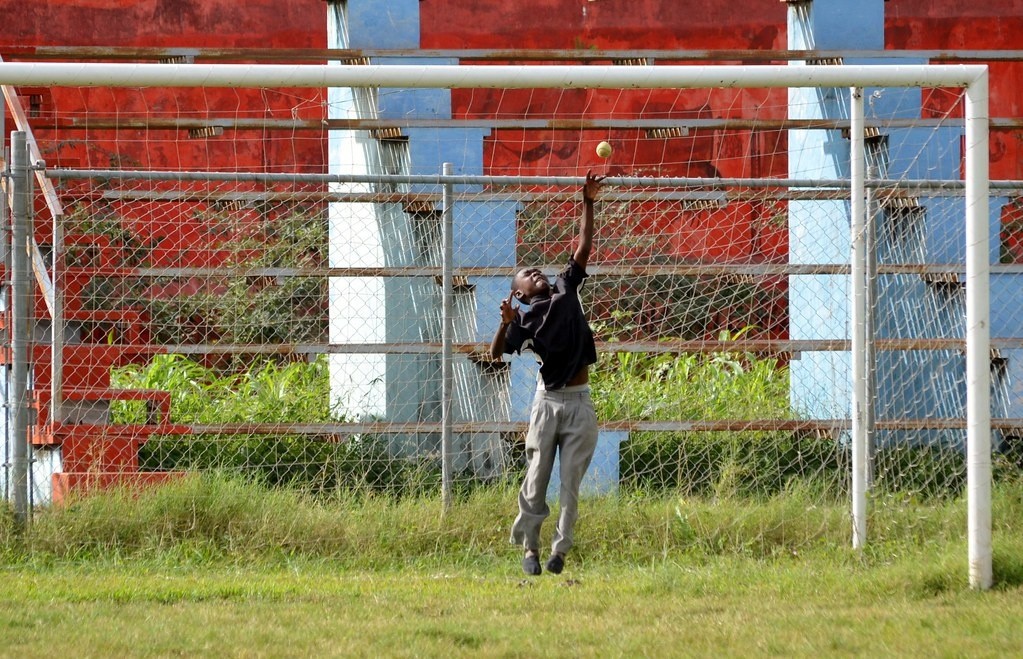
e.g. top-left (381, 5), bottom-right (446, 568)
top-left (492, 169), bottom-right (607, 576)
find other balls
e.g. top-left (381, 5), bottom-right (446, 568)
top-left (595, 140), bottom-right (612, 158)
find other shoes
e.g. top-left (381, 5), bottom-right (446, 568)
top-left (547, 554), bottom-right (564, 576)
top-left (523, 548), bottom-right (542, 576)
